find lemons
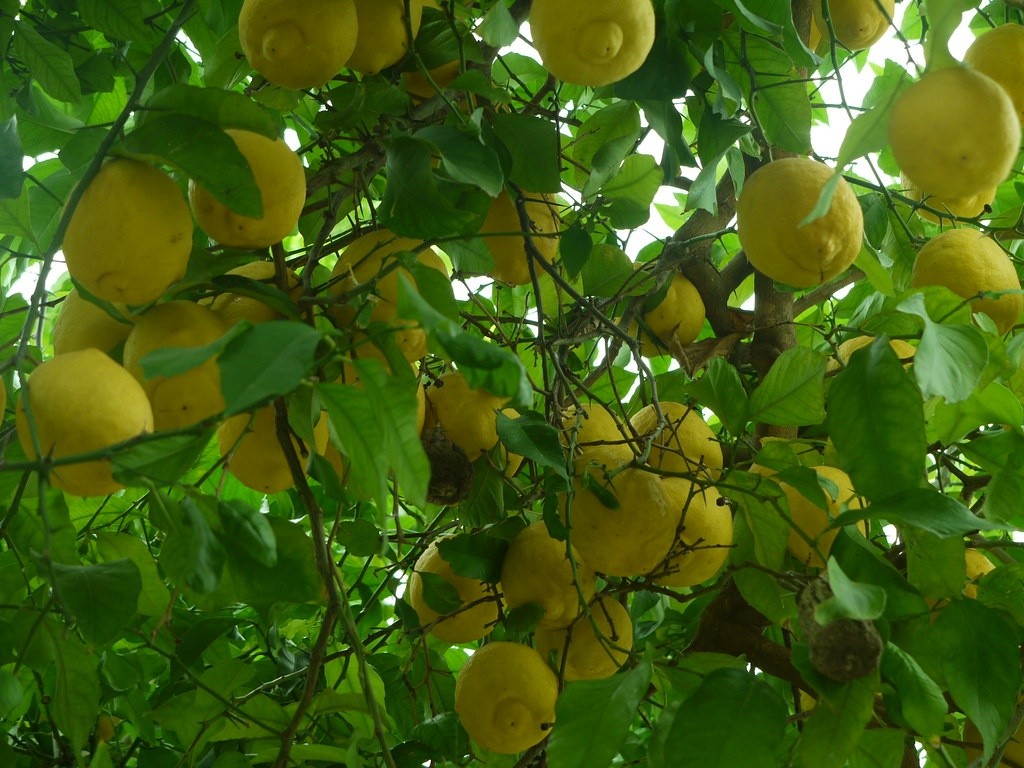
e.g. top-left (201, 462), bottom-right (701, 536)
top-left (16, 0), bottom-right (1024, 755)
